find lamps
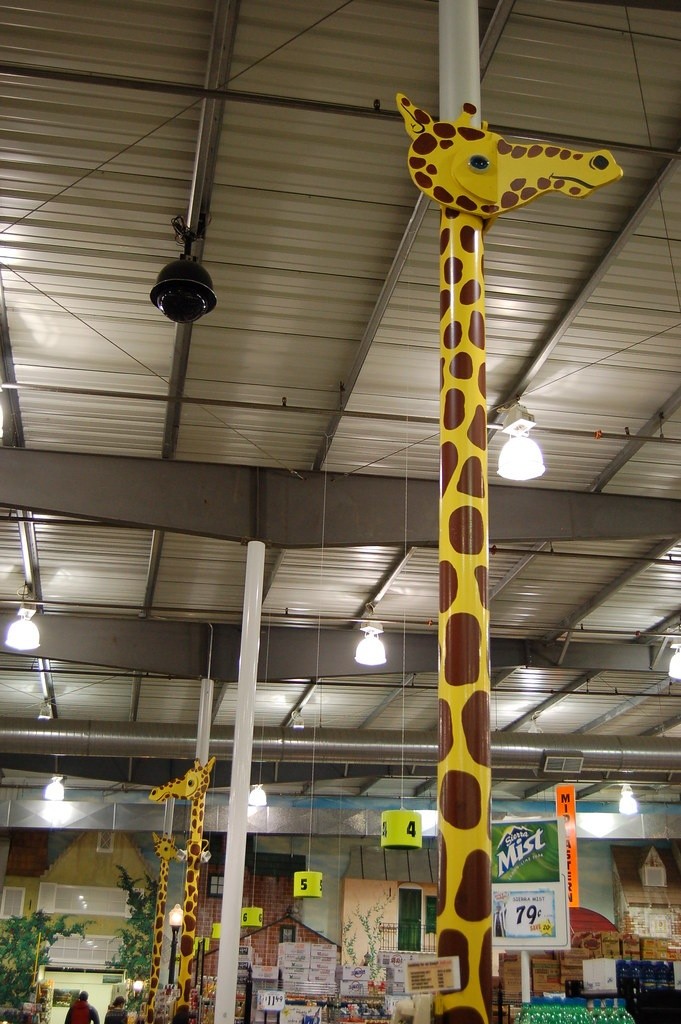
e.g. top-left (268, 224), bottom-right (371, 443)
top-left (355, 622), bottom-right (387, 666)
top-left (4, 604), bottom-right (40, 652)
top-left (496, 399), bottom-right (546, 481)
top-left (668, 640), bottom-right (681, 679)
top-left (249, 786), bottom-right (268, 807)
top-left (619, 785), bottom-right (638, 815)
top-left (44, 777), bottom-right (66, 800)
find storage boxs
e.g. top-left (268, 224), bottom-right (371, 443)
top-left (561, 966), bottom-right (583, 984)
top-left (601, 932), bottom-right (620, 954)
top-left (656, 949), bottom-right (668, 958)
top-left (571, 932), bottom-right (602, 957)
top-left (234, 941), bottom-right (436, 1024)
top-left (620, 933), bottom-right (640, 951)
top-left (499, 953), bottom-right (522, 974)
top-left (491, 977), bottom-right (498, 1004)
top-left (667, 949), bottom-right (681, 959)
top-left (604, 954), bottom-right (613, 959)
top-left (499, 973), bottom-right (521, 991)
top-left (613, 955), bottom-right (622, 959)
top-left (623, 951), bottom-right (631, 960)
top-left (502, 990), bottom-right (522, 1005)
top-left (640, 937), bottom-right (655, 948)
top-left (641, 948), bottom-right (655, 959)
top-left (532, 954), bottom-right (561, 990)
top-left (655, 938), bottom-right (669, 948)
top-left (631, 951), bottom-right (640, 960)
top-left (560, 948), bottom-right (589, 966)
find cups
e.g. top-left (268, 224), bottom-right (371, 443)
top-left (565, 979), bottom-right (584, 997)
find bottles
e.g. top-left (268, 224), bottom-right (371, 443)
top-left (195, 974), bottom-right (217, 1024)
top-left (616, 960), bottom-right (675, 1009)
top-left (236, 990), bottom-right (246, 1018)
top-left (286, 992), bottom-right (341, 1024)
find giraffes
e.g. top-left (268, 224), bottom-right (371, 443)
top-left (394, 95), bottom-right (625, 1024)
top-left (144, 756), bottom-right (218, 1024)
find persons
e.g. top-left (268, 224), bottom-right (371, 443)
top-left (65, 991), bottom-right (100, 1024)
top-left (104, 996), bottom-right (128, 1024)
top-left (172, 1004), bottom-right (190, 1024)
